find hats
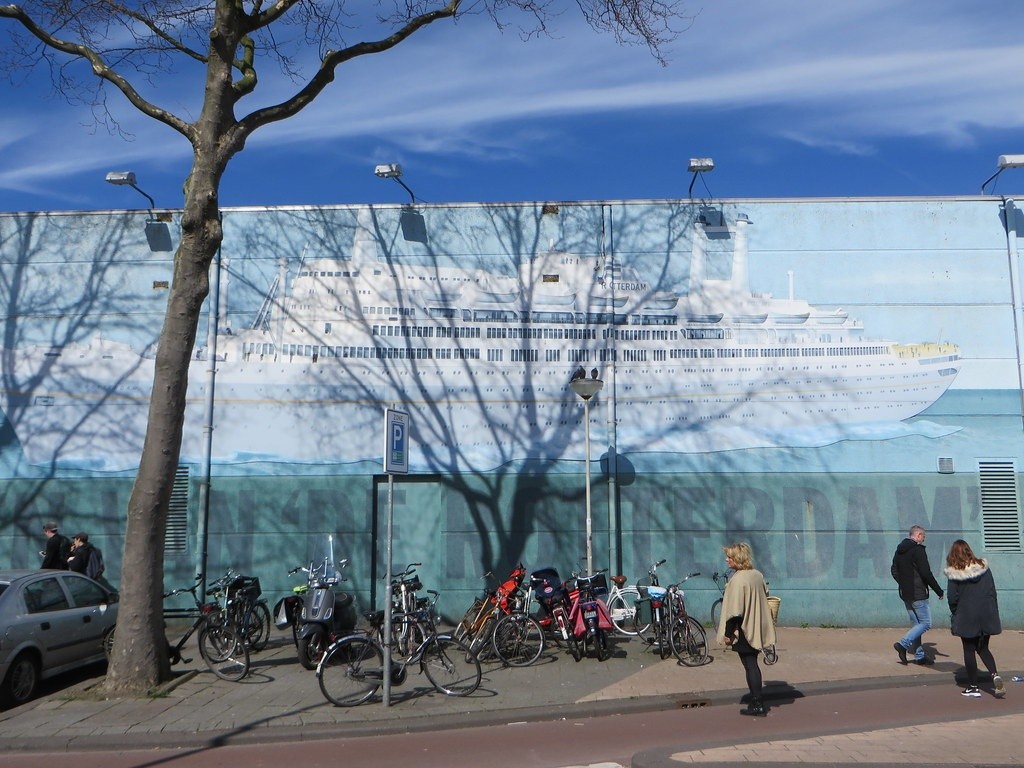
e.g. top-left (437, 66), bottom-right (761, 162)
top-left (43, 521), bottom-right (58, 532)
top-left (71, 532), bottom-right (88, 543)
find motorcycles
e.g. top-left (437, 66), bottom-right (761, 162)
top-left (293, 529), bottom-right (357, 671)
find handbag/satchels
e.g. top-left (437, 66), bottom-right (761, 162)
top-left (767, 596), bottom-right (781, 627)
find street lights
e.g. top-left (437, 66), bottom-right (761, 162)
top-left (569, 379), bottom-right (604, 576)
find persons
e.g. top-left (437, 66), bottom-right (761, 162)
top-left (944, 540), bottom-right (1006, 697)
top-left (891, 525), bottom-right (944, 665)
top-left (40, 522), bottom-right (94, 594)
top-left (716, 542), bottom-right (776, 716)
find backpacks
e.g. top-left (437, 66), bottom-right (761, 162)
top-left (85, 547), bottom-right (105, 580)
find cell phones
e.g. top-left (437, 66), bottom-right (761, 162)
top-left (39, 551), bottom-right (46, 557)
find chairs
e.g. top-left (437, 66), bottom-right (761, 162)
top-left (24, 589), bottom-right (69, 614)
top-left (530, 567), bottom-right (569, 609)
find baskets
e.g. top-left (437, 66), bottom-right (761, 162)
top-left (391, 575), bottom-right (419, 595)
top-left (636, 576), bottom-right (651, 598)
top-left (577, 574), bottom-right (607, 597)
top-left (226, 576), bottom-right (261, 600)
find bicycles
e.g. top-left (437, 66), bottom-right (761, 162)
top-left (315, 590), bottom-right (483, 708)
top-left (383, 563), bottom-right (431, 662)
top-left (530, 553), bottom-right (654, 662)
top-left (635, 559), bottom-right (709, 667)
top-left (453, 563), bottom-right (545, 667)
top-left (711, 566), bottom-right (769, 646)
top-left (103, 572), bottom-right (250, 683)
top-left (199, 567), bottom-right (270, 658)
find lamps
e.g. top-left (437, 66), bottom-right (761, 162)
top-left (374, 163), bottom-right (414, 204)
top-left (981, 154), bottom-right (1024, 195)
top-left (105, 171), bottom-right (154, 210)
top-left (687, 158), bottom-right (714, 199)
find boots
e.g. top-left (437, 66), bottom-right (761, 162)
top-left (740, 696), bottom-right (765, 716)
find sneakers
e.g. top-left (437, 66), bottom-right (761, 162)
top-left (961, 685), bottom-right (981, 697)
top-left (907, 645), bottom-right (922, 654)
top-left (991, 673), bottom-right (1007, 698)
top-left (894, 642), bottom-right (908, 665)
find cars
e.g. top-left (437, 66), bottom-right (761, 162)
top-left (0, 568), bottom-right (119, 706)
